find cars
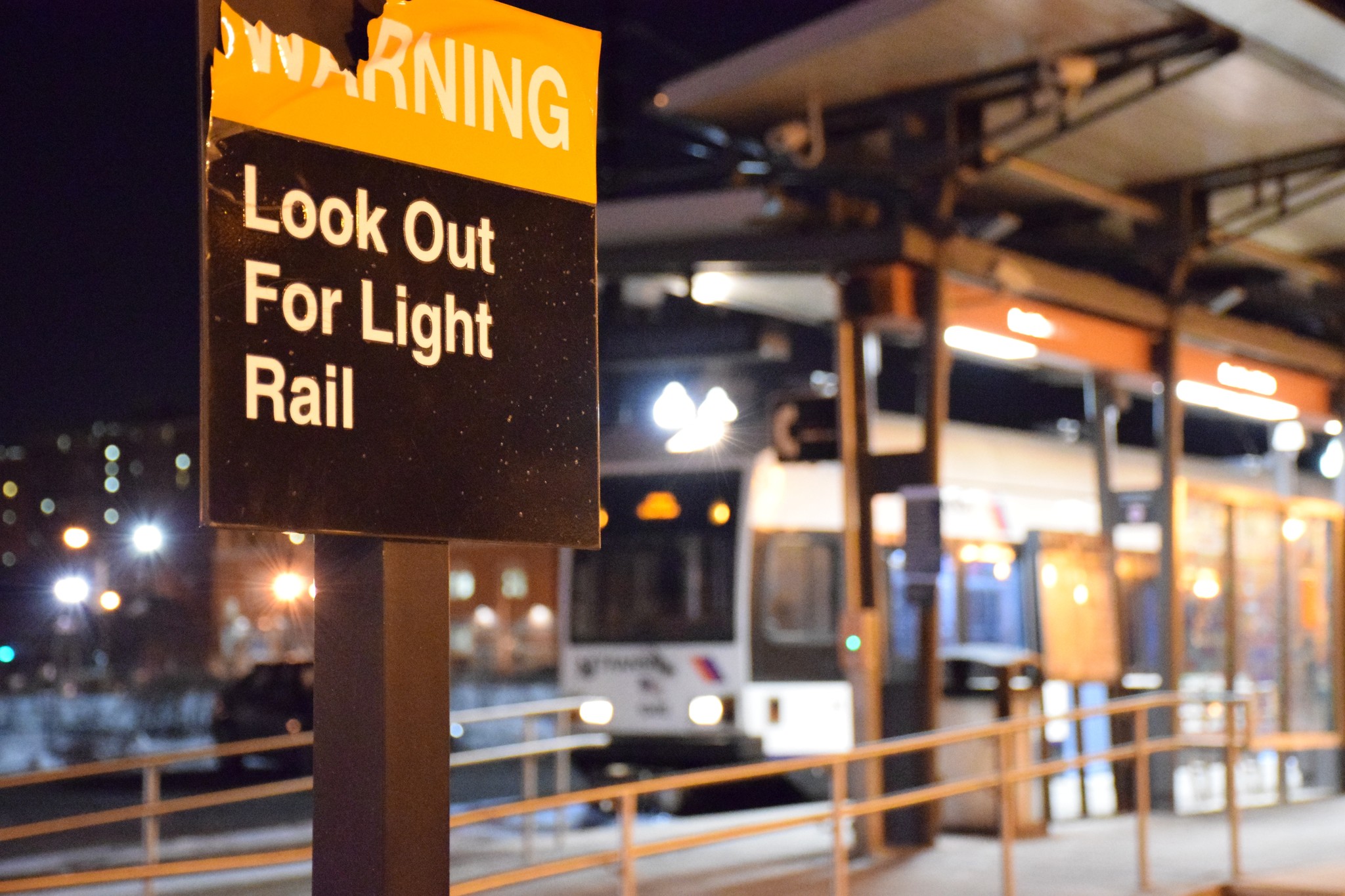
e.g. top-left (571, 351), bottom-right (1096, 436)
top-left (209, 661), bottom-right (314, 776)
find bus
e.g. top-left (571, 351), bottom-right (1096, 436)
top-left (557, 452), bottom-right (1343, 801)
